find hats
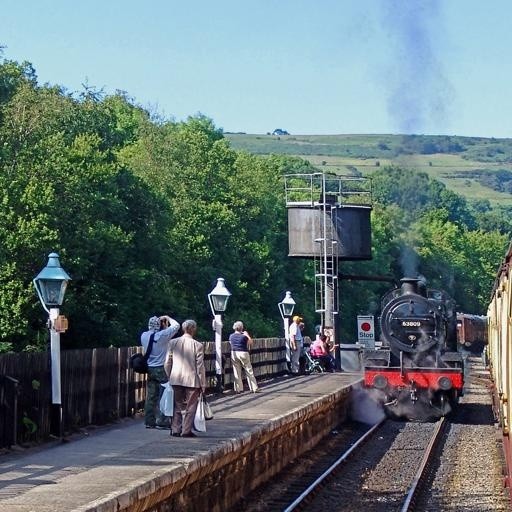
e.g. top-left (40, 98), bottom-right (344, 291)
top-left (293, 316), bottom-right (303, 321)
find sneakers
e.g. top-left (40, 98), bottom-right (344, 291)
top-left (172, 433), bottom-right (180, 437)
top-left (182, 431), bottom-right (197, 438)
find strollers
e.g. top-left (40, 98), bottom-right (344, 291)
top-left (303, 347), bottom-right (332, 374)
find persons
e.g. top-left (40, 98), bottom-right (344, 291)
top-left (138, 313), bottom-right (344, 439)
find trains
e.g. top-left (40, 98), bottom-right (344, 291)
top-left (362, 276), bottom-right (489, 420)
top-left (486, 242), bottom-right (510, 497)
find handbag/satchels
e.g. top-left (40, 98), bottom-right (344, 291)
top-left (203, 400), bottom-right (213, 421)
top-left (194, 400), bottom-right (206, 432)
top-left (130, 353), bottom-right (149, 374)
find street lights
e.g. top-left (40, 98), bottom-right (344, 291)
top-left (207, 277), bottom-right (232, 398)
top-left (277, 291), bottom-right (296, 374)
top-left (32, 253), bottom-right (72, 442)
